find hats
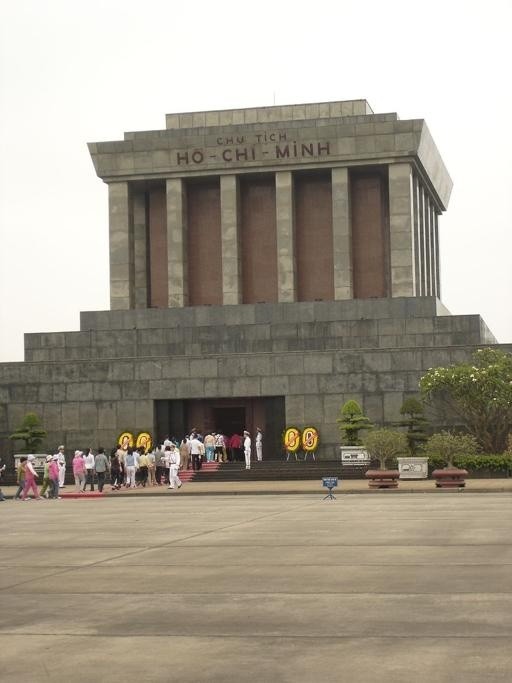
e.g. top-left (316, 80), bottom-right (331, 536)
top-left (75, 451), bottom-right (83, 457)
top-left (46, 455), bottom-right (53, 461)
top-left (257, 428), bottom-right (261, 432)
top-left (244, 431), bottom-right (250, 435)
top-left (53, 455), bottom-right (59, 461)
top-left (58, 446), bottom-right (64, 450)
top-left (171, 445), bottom-right (176, 447)
top-left (28, 455), bottom-right (35, 461)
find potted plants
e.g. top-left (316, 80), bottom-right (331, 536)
top-left (335, 399), bottom-right (371, 466)
top-left (8, 411), bottom-right (48, 473)
top-left (425, 429), bottom-right (482, 488)
top-left (364, 428), bottom-right (409, 489)
top-left (396, 397), bottom-right (430, 479)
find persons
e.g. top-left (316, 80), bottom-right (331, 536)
top-left (255, 426), bottom-right (263, 462)
top-left (243, 430), bottom-right (252, 471)
top-left (0, 456), bottom-right (8, 502)
top-left (12, 424), bottom-right (245, 500)
